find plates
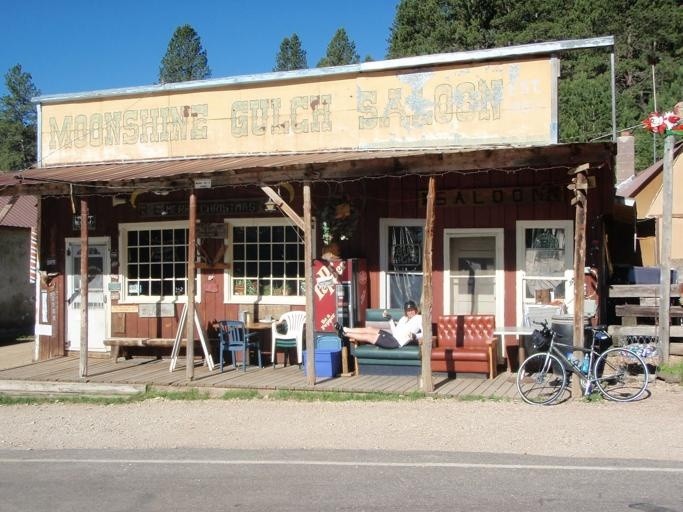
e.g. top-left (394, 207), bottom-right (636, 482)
top-left (259, 319), bottom-right (276, 324)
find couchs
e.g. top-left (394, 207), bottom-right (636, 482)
top-left (350, 307), bottom-right (500, 379)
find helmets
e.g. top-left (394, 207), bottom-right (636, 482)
top-left (404, 300), bottom-right (418, 315)
top-left (531, 329), bottom-right (550, 351)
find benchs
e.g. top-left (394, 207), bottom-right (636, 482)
top-left (102, 336), bottom-right (218, 367)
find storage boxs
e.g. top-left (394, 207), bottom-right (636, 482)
top-left (302, 348), bottom-right (343, 378)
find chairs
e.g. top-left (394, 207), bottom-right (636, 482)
top-left (271, 311), bottom-right (306, 369)
top-left (218, 320), bottom-right (264, 373)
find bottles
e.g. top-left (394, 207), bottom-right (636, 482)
top-left (582, 352), bottom-right (590, 376)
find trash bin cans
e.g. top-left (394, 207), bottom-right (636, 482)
top-left (551, 315), bottom-right (591, 375)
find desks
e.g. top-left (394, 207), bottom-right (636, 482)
top-left (493, 324), bottom-right (553, 384)
top-left (211, 319), bottom-right (276, 331)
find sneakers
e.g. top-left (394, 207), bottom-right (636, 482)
top-left (334, 322), bottom-right (344, 337)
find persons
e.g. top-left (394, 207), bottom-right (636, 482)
top-left (333, 300), bottom-right (424, 349)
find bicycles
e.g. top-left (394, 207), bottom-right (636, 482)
top-left (512, 315), bottom-right (648, 408)
